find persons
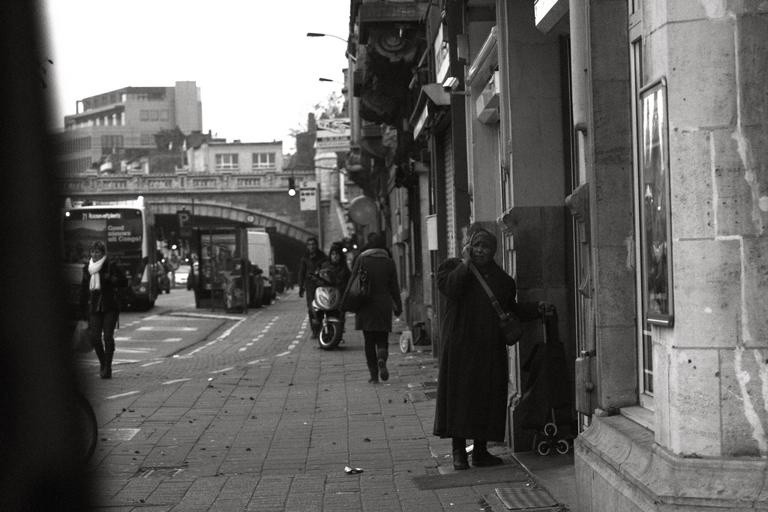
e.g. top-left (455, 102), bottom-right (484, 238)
top-left (81, 240), bottom-right (120, 379)
top-left (310, 242), bottom-right (351, 336)
top-left (432, 227), bottom-right (555, 470)
top-left (297, 237), bottom-right (328, 339)
top-left (349, 232), bottom-right (402, 384)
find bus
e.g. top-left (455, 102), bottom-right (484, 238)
top-left (60, 195), bottom-right (160, 314)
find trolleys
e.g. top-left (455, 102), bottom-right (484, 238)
top-left (517, 304), bottom-right (574, 458)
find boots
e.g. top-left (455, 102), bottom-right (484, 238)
top-left (452, 436), bottom-right (470, 470)
top-left (378, 350), bottom-right (389, 380)
top-left (100, 336), bottom-right (114, 379)
top-left (471, 441), bottom-right (502, 465)
top-left (366, 360), bottom-right (379, 383)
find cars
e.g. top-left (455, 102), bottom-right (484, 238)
top-left (157, 263), bottom-right (201, 292)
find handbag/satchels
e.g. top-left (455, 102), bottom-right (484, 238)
top-left (95, 290), bottom-right (122, 313)
top-left (340, 260), bottom-right (370, 311)
top-left (497, 311), bottom-right (524, 345)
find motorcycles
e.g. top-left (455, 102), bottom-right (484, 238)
top-left (310, 266), bottom-right (346, 351)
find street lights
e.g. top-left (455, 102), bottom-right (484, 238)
top-left (306, 31), bottom-right (354, 144)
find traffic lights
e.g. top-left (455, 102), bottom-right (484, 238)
top-left (288, 178), bottom-right (296, 196)
